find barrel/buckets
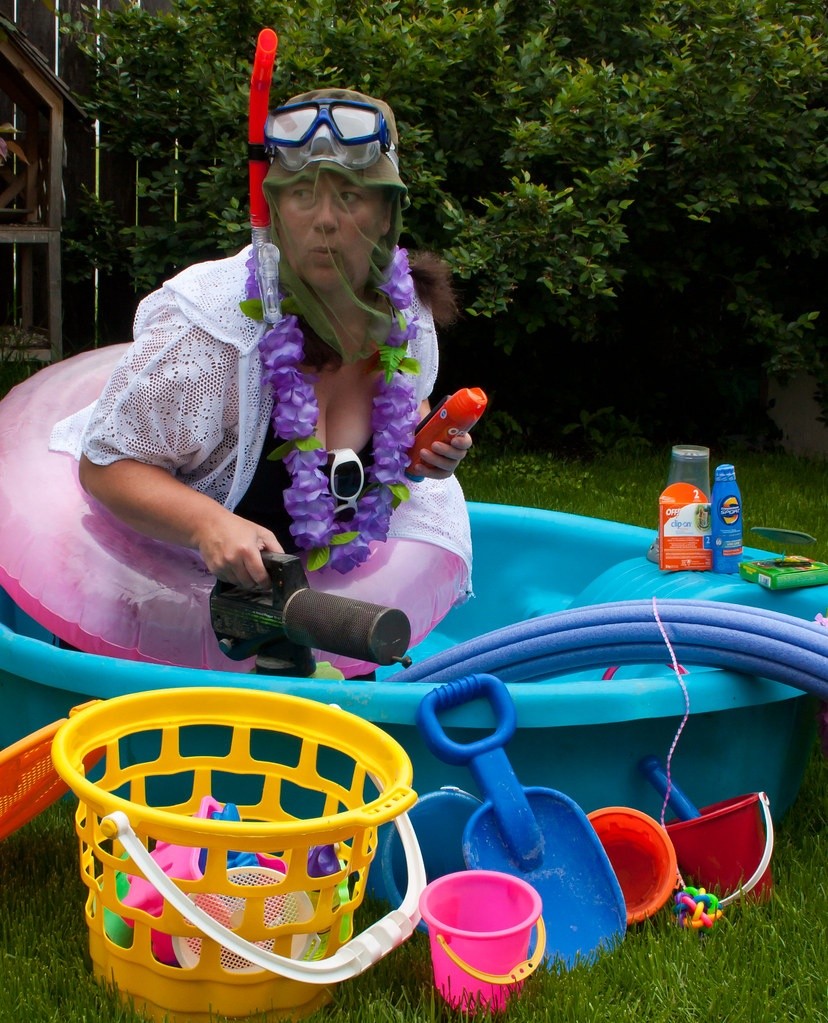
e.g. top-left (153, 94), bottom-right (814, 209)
top-left (365, 787), bottom-right (485, 931)
top-left (662, 791), bottom-right (773, 907)
top-left (419, 870), bottom-right (546, 1015)
top-left (587, 807), bottom-right (681, 924)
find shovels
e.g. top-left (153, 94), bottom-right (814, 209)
top-left (415, 674), bottom-right (629, 978)
top-left (641, 758), bottom-right (701, 822)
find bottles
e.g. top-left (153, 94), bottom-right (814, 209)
top-left (404, 387), bottom-right (487, 482)
top-left (667, 445), bottom-right (711, 502)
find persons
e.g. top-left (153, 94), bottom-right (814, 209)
top-left (47, 89), bottom-right (473, 682)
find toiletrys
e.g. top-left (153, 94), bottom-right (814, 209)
top-left (712, 462), bottom-right (744, 574)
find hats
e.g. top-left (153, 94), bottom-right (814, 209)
top-left (262, 88), bottom-right (410, 224)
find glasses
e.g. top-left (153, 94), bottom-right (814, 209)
top-left (328, 448), bottom-right (364, 524)
top-left (263, 97), bottom-right (388, 148)
